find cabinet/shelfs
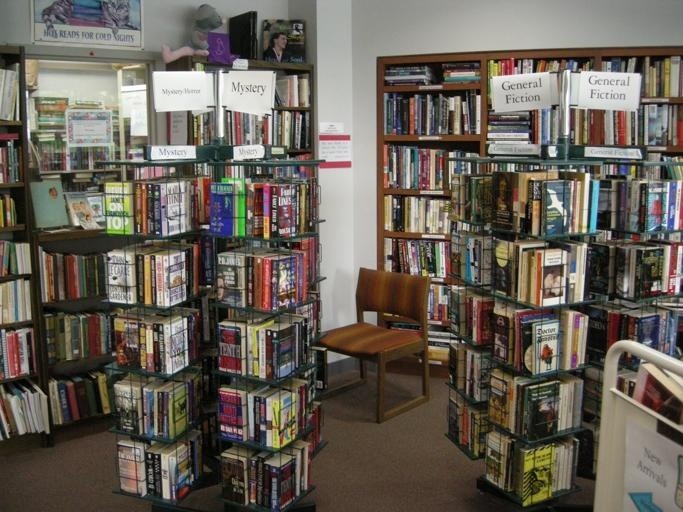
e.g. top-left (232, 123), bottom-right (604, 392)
top-left (444, 64), bottom-right (638, 504)
top-left (376, 48), bottom-right (682, 375)
top-left (596, 337), bottom-right (683, 512)
top-left (0, 45), bottom-right (328, 512)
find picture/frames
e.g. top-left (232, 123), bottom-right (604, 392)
top-left (30, 1), bottom-right (145, 51)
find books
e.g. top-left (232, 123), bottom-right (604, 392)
top-left (381, 58), bottom-right (682, 478)
top-left (445, 169), bottom-right (601, 507)
top-left (104, 175), bottom-right (325, 510)
top-left (1, 11), bottom-right (332, 442)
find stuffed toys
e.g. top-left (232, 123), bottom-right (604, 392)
top-left (183, 3), bottom-right (224, 50)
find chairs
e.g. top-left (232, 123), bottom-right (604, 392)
top-left (315, 268), bottom-right (432, 424)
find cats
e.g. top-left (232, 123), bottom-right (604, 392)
top-left (100, 0), bottom-right (141, 35)
top-left (40, 0), bottom-right (74, 32)
